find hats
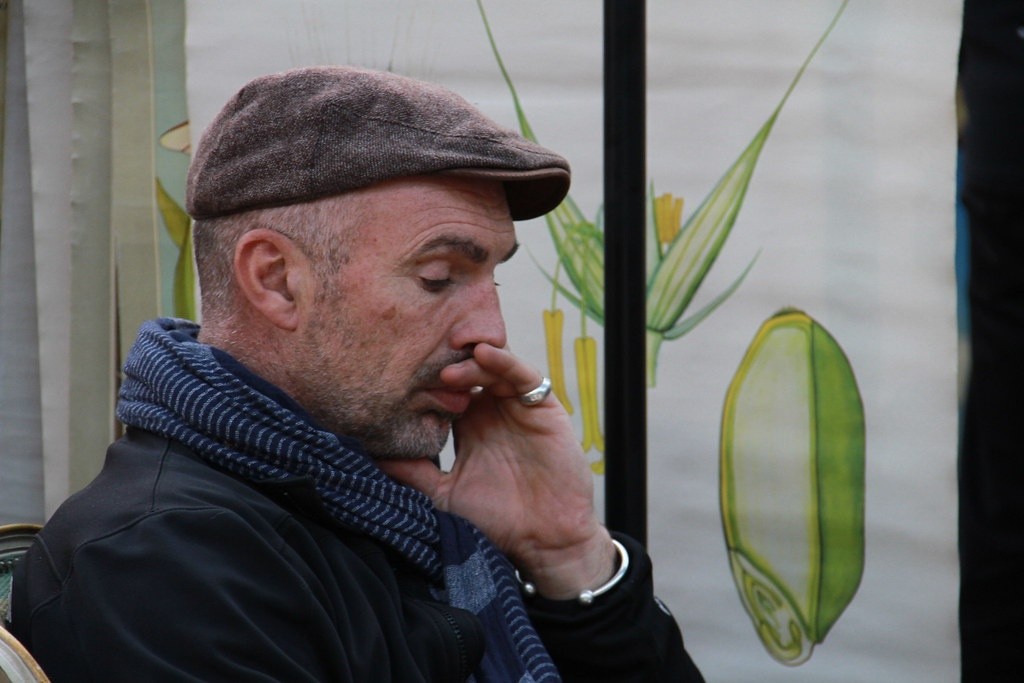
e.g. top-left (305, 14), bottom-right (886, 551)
top-left (184, 67), bottom-right (570, 221)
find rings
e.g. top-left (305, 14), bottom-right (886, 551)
top-left (517, 376), bottom-right (552, 406)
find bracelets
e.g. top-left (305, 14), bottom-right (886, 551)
top-left (514, 539), bottom-right (630, 605)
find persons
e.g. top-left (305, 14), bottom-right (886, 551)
top-left (9, 63), bottom-right (713, 683)
